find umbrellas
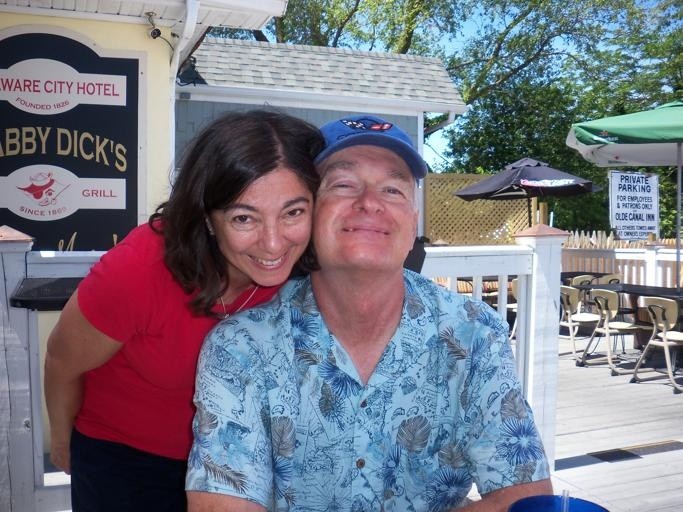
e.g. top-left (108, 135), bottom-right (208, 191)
top-left (565, 97), bottom-right (683, 292)
top-left (455, 158), bottom-right (604, 228)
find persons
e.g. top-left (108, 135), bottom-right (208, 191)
top-left (43, 110), bottom-right (327, 512)
top-left (184, 115), bottom-right (554, 511)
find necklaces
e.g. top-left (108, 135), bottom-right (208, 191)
top-left (220, 284), bottom-right (259, 320)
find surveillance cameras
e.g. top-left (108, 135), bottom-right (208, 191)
top-left (146, 28), bottom-right (161, 39)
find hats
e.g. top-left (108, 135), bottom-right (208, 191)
top-left (312, 113), bottom-right (428, 181)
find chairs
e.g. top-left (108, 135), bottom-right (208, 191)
top-left (457, 271), bottom-right (682, 394)
